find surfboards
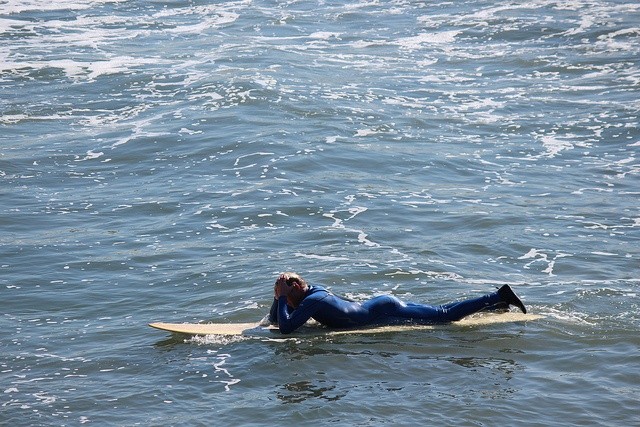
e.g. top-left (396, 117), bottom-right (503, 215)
top-left (148, 309), bottom-right (548, 338)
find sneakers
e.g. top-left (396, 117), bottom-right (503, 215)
top-left (497, 284), bottom-right (527, 314)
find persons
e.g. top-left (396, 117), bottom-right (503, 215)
top-left (269, 272), bottom-right (527, 335)
